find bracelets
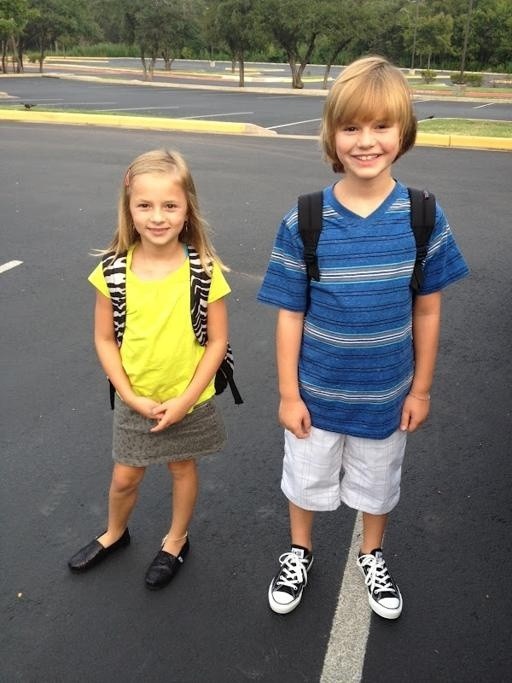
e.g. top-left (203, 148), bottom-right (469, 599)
top-left (409, 392), bottom-right (431, 401)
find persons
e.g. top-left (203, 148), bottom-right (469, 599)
top-left (256, 55), bottom-right (471, 619)
top-left (68, 149), bottom-right (232, 591)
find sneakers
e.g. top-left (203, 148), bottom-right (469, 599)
top-left (268, 544), bottom-right (314, 614)
top-left (357, 548), bottom-right (403, 620)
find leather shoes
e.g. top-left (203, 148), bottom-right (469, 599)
top-left (145, 534), bottom-right (190, 591)
top-left (68, 527), bottom-right (130, 573)
